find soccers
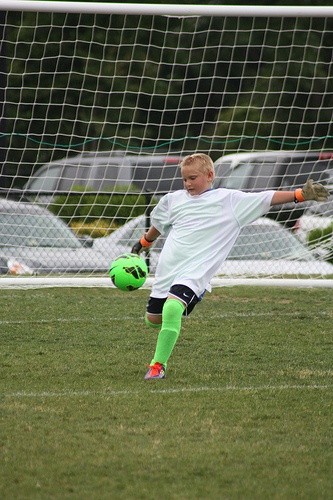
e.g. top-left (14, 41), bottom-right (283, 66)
top-left (108, 252), bottom-right (149, 292)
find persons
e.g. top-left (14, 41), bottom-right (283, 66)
top-left (131, 153), bottom-right (330, 381)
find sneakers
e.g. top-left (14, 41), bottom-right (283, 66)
top-left (144, 362), bottom-right (166, 379)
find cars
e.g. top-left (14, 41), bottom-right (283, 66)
top-left (25, 151), bottom-right (186, 202)
top-left (210, 148), bottom-right (332, 225)
top-left (91, 211), bottom-right (333, 276)
top-left (292, 200), bottom-right (333, 262)
top-left (0, 197), bottom-right (110, 276)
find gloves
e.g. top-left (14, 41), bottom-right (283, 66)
top-left (131, 233), bottom-right (155, 256)
top-left (294, 180), bottom-right (330, 204)
